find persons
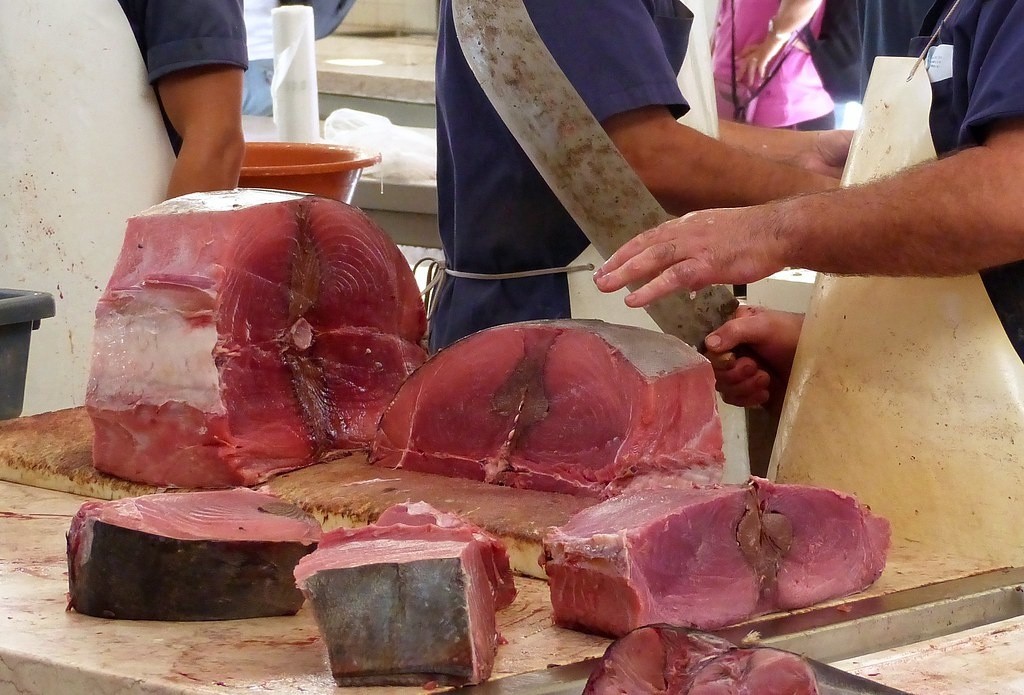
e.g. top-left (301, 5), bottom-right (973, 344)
top-left (240, 0), bottom-right (358, 118)
top-left (1, 0), bottom-right (244, 420)
top-left (709, 0), bottom-right (837, 136)
top-left (588, 1), bottom-right (1023, 567)
top-left (429, 0), bottom-right (857, 357)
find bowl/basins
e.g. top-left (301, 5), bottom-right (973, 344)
top-left (237, 141), bottom-right (382, 206)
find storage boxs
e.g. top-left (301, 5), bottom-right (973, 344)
top-left (0, 289), bottom-right (55, 421)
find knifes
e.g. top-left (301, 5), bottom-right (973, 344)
top-left (450, 0), bottom-right (785, 411)
